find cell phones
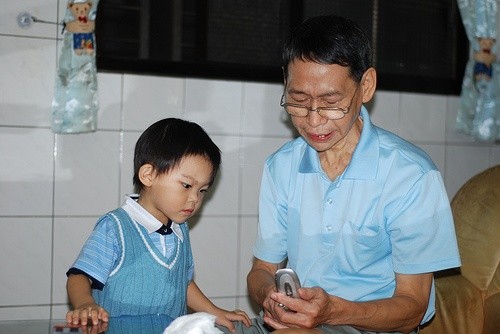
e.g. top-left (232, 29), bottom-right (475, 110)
top-left (275, 268), bottom-right (302, 312)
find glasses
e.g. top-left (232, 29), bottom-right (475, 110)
top-left (279, 77), bottom-right (361, 121)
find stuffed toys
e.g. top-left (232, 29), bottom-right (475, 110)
top-left (64, 0), bottom-right (95, 57)
top-left (473, 36), bottom-right (497, 93)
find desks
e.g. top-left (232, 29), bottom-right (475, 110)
top-left (0, 314), bottom-right (175, 334)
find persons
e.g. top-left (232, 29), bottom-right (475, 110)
top-left (65, 118), bottom-right (252, 334)
top-left (214, 14), bottom-right (463, 334)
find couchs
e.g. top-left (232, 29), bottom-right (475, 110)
top-left (421, 164), bottom-right (500, 334)
